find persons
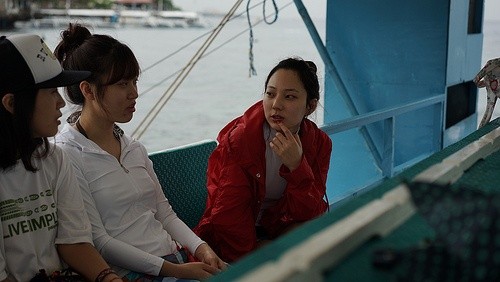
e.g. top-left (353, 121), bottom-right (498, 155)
top-left (188, 58), bottom-right (333, 277)
top-left (0, 33), bottom-right (122, 282)
top-left (48, 23), bottom-right (232, 282)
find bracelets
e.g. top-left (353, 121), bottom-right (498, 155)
top-left (95, 268), bottom-right (120, 282)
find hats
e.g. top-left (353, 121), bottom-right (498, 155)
top-left (0, 33), bottom-right (92, 89)
